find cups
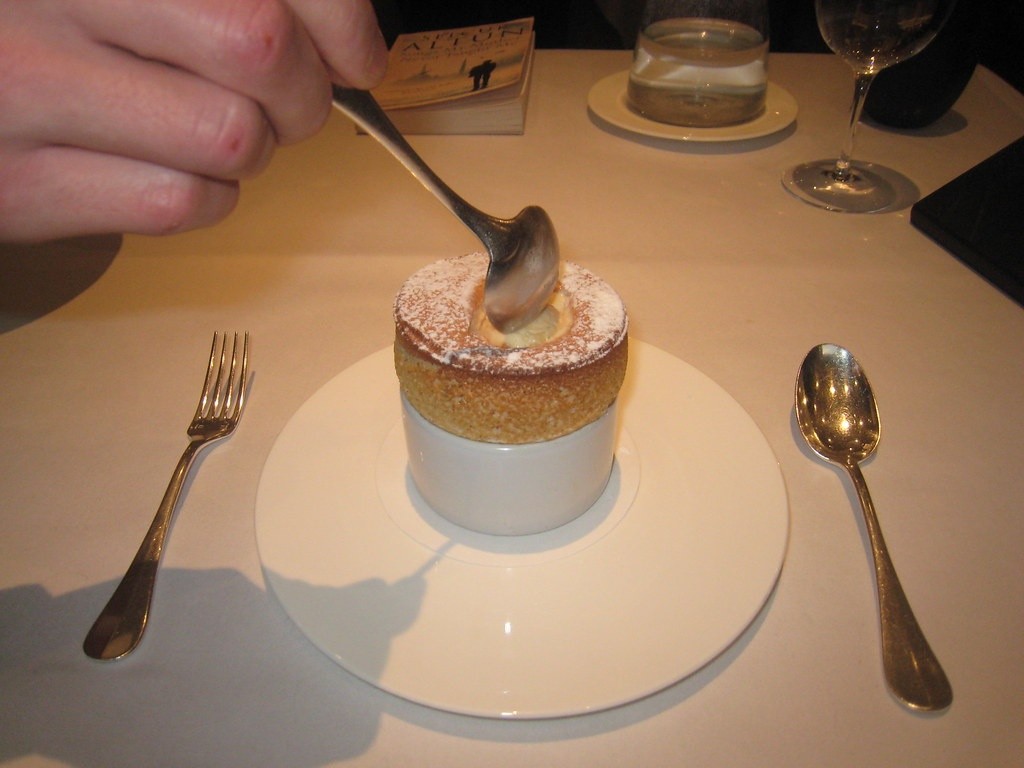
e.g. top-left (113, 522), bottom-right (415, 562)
top-left (626, 0), bottom-right (772, 126)
top-left (863, 0), bottom-right (987, 129)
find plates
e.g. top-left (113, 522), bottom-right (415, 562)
top-left (587, 70), bottom-right (799, 141)
top-left (253, 335), bottom-right (788, 716)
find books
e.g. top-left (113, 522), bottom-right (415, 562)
top-left (355, 17), bottom-right (536, 137)
top-left (909, 131), bottom-right (1024, 307)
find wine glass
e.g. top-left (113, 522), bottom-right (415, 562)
top-left (781, 0), bottom-right (944, 213)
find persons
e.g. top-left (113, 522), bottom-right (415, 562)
top-left (0, 0), bottom-right (388, 242)
top-left (468, 60), bottom-right (496, 92)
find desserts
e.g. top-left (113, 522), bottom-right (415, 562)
top-left (391, 249), bottom-right (630, 444)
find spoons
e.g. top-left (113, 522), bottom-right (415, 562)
top-left (794, 341), bottom-right (953, 714)
top-left (333, 84), bottom-right (559, 334)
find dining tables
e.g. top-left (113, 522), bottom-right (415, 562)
top-left (0, 49), bottom-right (1024, 768)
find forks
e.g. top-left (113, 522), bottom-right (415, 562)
top-left (80, 330), bottom-right (251, 661)
